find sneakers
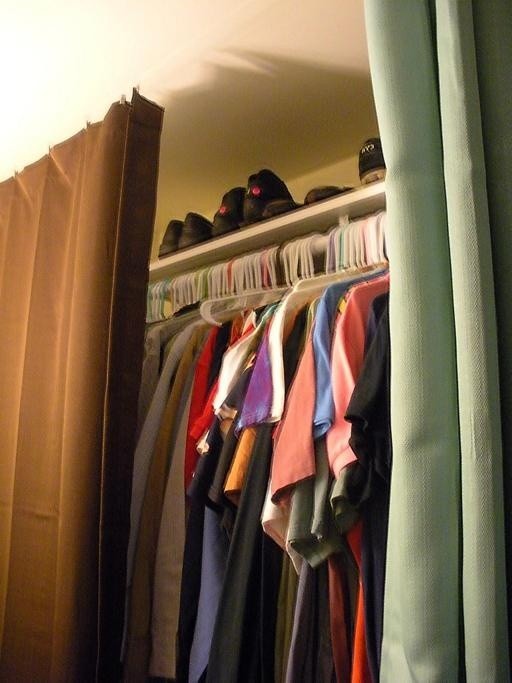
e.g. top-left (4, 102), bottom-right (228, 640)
top-left (158, 137), bottom-right (387, 258)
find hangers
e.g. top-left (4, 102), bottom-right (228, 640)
top-left (145, 209), bottom-right (389, 330)
